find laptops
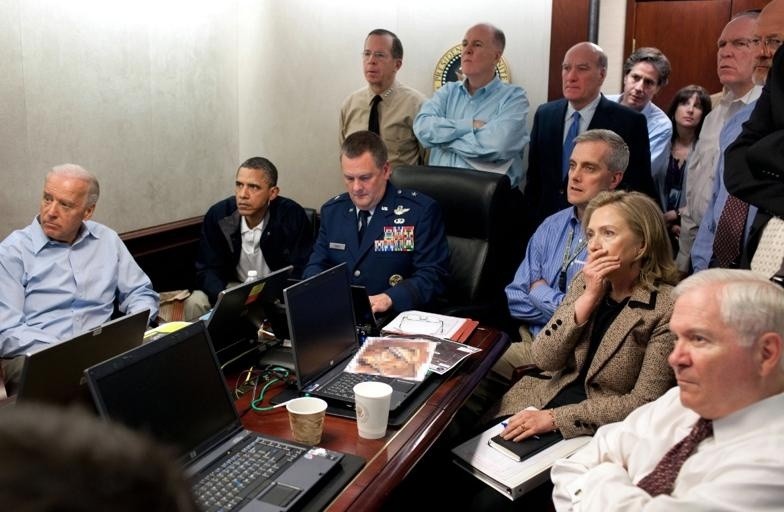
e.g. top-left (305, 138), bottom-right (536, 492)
top-left (0, 309), bottom-right (149, 420)
top-left (184, 265), bottom-right (294, 343)
top-left (284, 262), bottom-right (435, 412)
top-left (288, 277), bottom-right (393, 335)
top-left (84, 320), bottom-right (345, 511)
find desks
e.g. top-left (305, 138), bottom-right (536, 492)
top-left (220, 310), bottom-right (513, 512)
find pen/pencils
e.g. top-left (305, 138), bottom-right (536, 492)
top-left (501, 422), bottom-right (540, 440)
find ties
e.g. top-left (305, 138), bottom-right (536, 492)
top-left (638, 418), bottom-right (713, 497)
top-left (752, 217), bottom-right (784, 280)
top-left (357, 210), bottom-right (370, 244)
top-left (561, 113), bottom-right (581, 179)
top-left (368, 95), bottom-right (383, 137)
top-left (712, 195), bottom-right (751, 269)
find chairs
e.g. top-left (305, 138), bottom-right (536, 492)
top-left (199, 192), bottom-right (319, 257)
top-left (384, 160), bottom-right (514, 328)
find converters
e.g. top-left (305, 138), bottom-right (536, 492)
top-left (259, 350), bottom-right (296, 375)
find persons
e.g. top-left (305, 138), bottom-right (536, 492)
top-left (1, 162), bottom-right (161, 398)
top-left (1, 397), bottom-right (212, 511)
top-left (182, 155), bottom-right (312, 321)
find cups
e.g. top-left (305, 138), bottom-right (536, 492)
top-left (353, 381), bottom-right (392, 440)
top-left (285, 396), bottom-right (328, 446)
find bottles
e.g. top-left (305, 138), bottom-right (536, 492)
top-left (243, 270), bottom-right (259, 285)
